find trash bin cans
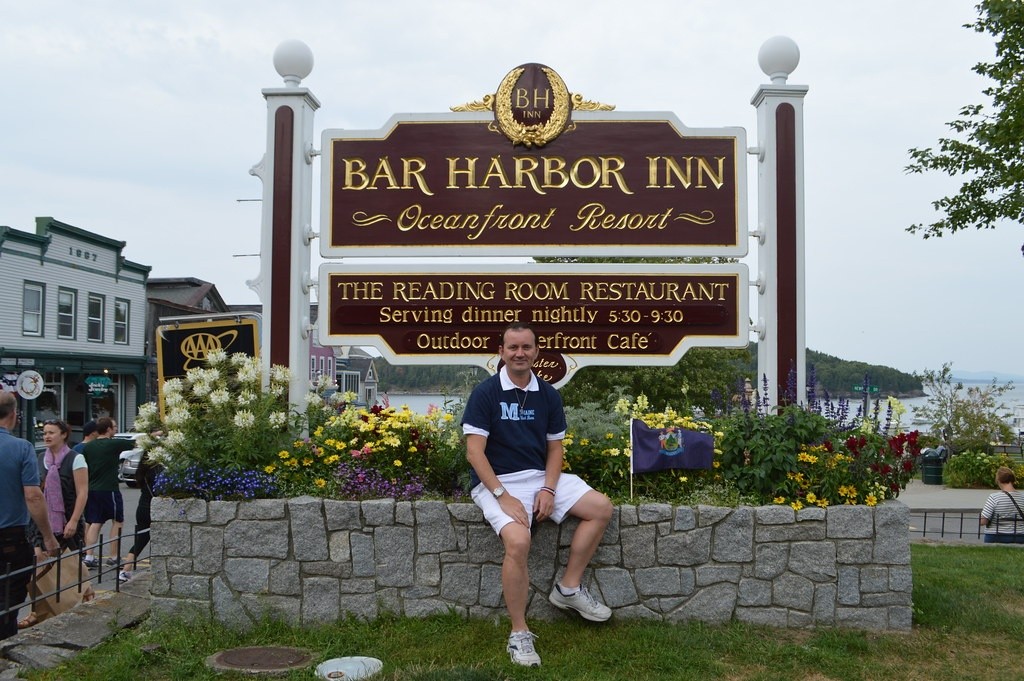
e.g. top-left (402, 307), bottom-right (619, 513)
top-left (920, 446), bottom-right (948, 485)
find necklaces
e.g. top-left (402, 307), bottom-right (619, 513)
top-left (513, 382), bottom-right (530, 415)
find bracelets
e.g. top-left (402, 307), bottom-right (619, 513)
top-left (541, 485), bottom-right (556, 496)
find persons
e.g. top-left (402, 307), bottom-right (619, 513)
top-left (460, 323), bottom-right (613, 667)
top-left (118, 443), bottom-right (166, 581)
top-left (73, 417), bottom-right (163, 569)
top-left (0, 390), bottom-right (62, 640)
top-left (980, 466), bottom-right (1024, 543)
top-left (18, 419), bottom-right (95, 629)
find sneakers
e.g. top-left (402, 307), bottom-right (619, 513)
top-left (119, 570), bottom-right (134, 582)
top-left (549, 582), bottom-right (612, 621)
top-left (507, 630), bottom-right (541, 669)
top-left (105, 557), bottom-right (123, 567)
top-left (82, 559), bottom-right (99, 570)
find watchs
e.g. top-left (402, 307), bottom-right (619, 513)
top-left (493, 487), bottom-right (506, 499)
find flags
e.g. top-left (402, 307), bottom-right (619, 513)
top-left (633, 418), bottom-right (714, 473)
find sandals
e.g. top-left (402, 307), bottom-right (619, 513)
top-left (18, 613), bottom-right (49, 628)
top-left (82, 592), bottom-right (95, 602)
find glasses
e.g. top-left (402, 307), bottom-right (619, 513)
top-left (44, 419), bottom-right (66, 432)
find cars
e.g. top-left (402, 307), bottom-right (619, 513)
top-left (112, 433), bottom-right (151, 489)
